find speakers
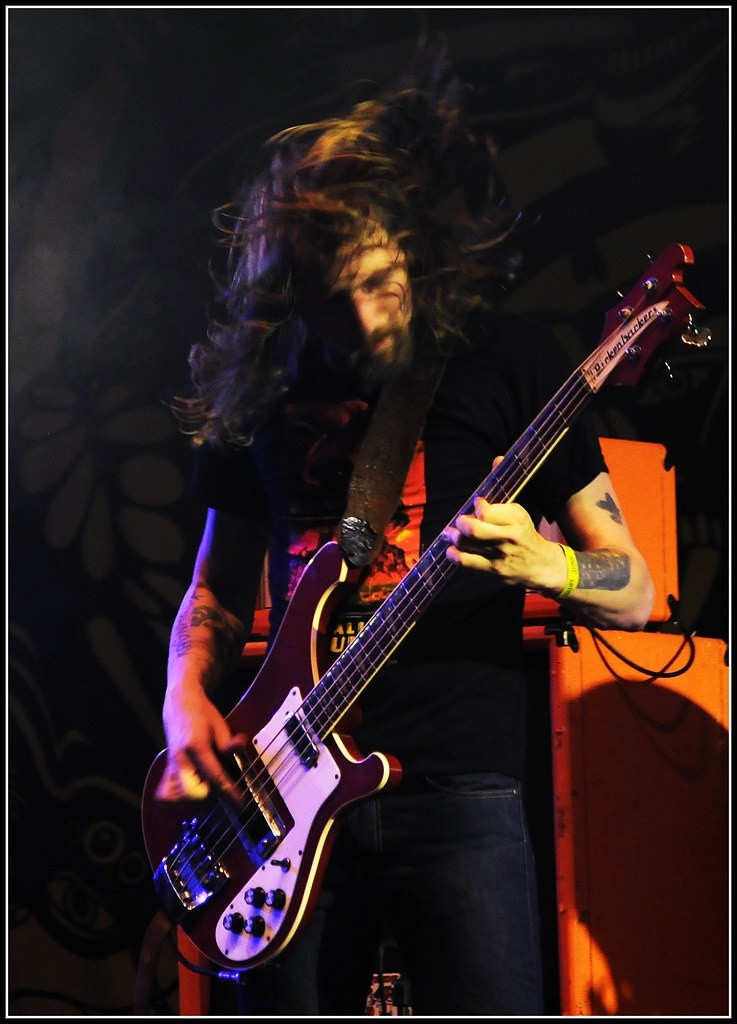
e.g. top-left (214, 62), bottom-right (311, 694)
top-left (521, 621), bottom-right (728, 1015)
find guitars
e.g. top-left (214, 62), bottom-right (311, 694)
top-left (138, 244), bottom-right (714, 969)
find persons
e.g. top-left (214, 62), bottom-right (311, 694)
top-left (153, 94), bottom-right (655, 1017)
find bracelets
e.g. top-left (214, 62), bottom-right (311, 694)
top-left (559, 541), bottom-right (580, 604)
